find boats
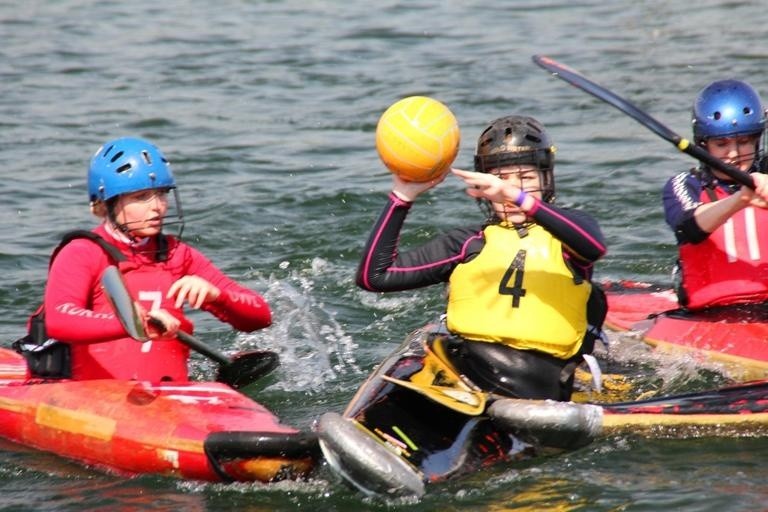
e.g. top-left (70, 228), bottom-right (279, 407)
top-left (0, 306), bottom-right (321, 486)
top-left (601, 276), bottom-right (768, 379)
top-left (487, 378), bottom-right (768, 451)
top-left (313, 280), bottom-right (610, 502)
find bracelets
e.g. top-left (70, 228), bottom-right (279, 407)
top-left (515, 189), bottom-right (529, 204)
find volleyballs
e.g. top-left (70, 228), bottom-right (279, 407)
top-left (376, 96), bottom-right (461, 181)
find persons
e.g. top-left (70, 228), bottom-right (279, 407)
top-left (355, 116), bottom-right (607, 397)
top-left (662, 78), bottom-right (767, 316)
top-left (44, 136), bottom-right (271, 382)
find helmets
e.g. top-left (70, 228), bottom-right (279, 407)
top-left (87, 136), bottom-right (177, 202)
top-left (474, 115), bottom-right (556, 172)
top-left (692, 79), bottom-right (768, 143)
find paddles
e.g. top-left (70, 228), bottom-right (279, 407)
top-left (99, 264), bottom-right (278, 391)
top-left (531, 55), bottom-right (755, 189)
top-left (380, 375), bottom-right (766, 416)
